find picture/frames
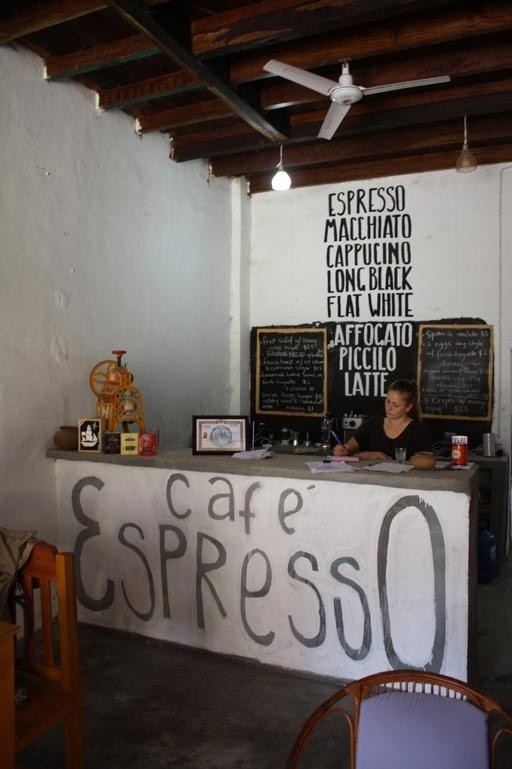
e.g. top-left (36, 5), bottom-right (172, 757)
top-left (77, 418), bottom-right (102, 453)
top-left (191, 415), bottom-right (250, 456)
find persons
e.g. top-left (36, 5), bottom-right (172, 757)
top-left (334, 378), bottom-right (429, 462)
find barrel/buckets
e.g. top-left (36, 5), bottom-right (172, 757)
top-left (477, 519), bottom-right (496, 584)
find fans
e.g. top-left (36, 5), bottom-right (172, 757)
top-left (263, 57), bottom-right (452, 143)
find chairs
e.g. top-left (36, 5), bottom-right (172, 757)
top-left (283, 669), bottom-right (512, 768)
top-left (4, 538), bottom-right (85, 769)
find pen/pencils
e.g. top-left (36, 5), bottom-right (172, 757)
top-left (368, 463), bottom-right (377, 466)
top-left (330, 429), bottom-right (348, 454)
top-left (323, 460), bottom-right (341, 464)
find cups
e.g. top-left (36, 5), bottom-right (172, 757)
top-left (483, 433), bottom-right (496, 457)
top-left (103, 432), bottom-right (121, 454)
top-left (451, 443), bottom-right (468, 466)
top-left (395, 448), bottom-right (406, 464)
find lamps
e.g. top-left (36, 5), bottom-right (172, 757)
top-left (453, 116), bottom-right (479, 175)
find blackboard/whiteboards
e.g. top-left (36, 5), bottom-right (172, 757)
top-left (256, 328), bottom-right (328, 418)
top-left (418, 324), bottom-right (495, 421)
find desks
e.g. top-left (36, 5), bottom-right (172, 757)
top-left (439, 455), bottom-right (509, 582)
